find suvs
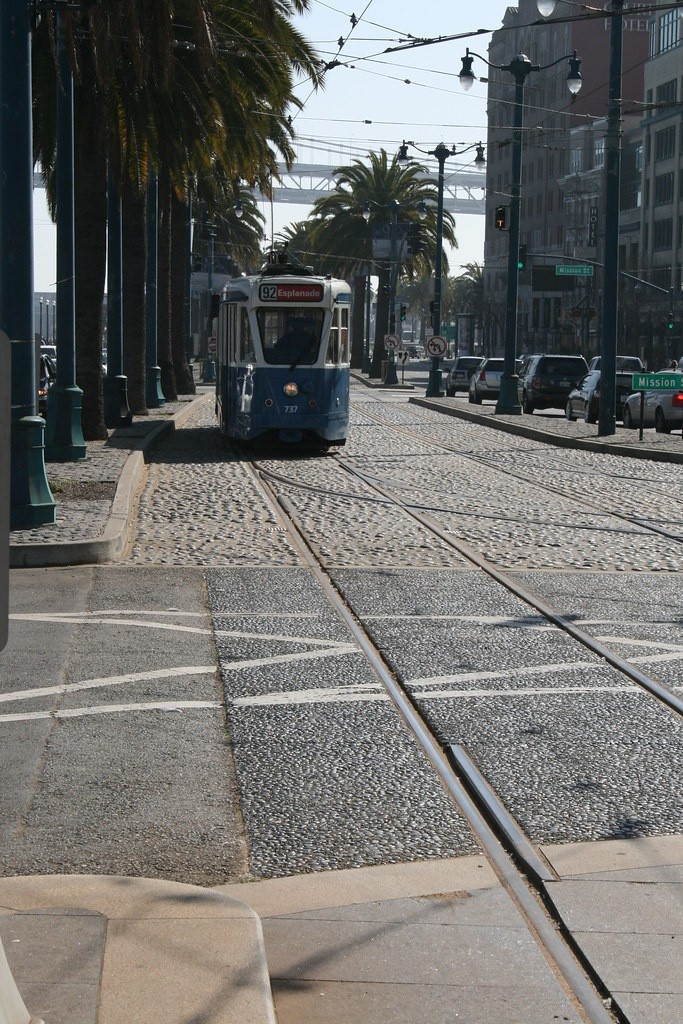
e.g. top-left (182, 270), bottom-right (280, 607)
top-left (407, 346), bottom-right (426, 360)
top-left (468, 357), bottom-right (524, 404)
top-left (517, 352), bottom-right (590, 414)
top-left (587, 356), bottom-right (645, 371)
top-left (444, 355), bottom-right (488, 397)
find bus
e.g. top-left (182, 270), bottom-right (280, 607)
top-left (214, 238), bottom-right (351, 452)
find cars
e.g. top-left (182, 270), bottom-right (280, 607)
top-left (39, 345), bottom-right (57, 419)
top-left (623, 369), bottom-right (683, 434)
top-left (564, 370), bottom-right (644, 425)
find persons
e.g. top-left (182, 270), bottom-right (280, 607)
top-left (271, 317), bottom-right (319, 364)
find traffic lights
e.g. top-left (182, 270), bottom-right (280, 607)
top-left (400, 304), bottom-right (407, 321)
top-left (495, 206), bottom-right (505, 228)
top-left (518, 244), bottom-right (526, 271)
top-left (669, 312), bottom-right (674, 329)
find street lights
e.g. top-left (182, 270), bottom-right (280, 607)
top-left (458, 47), bottom-right (583, 415)
top-left (398, 138), bottom-right (485, 397)
top-left (537, 0), bottom-right (683, 438)
top-left (362, 197), bottom-right (427, 386)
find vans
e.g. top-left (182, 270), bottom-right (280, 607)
top-left (402, 330), bottom-right (417, 345)
